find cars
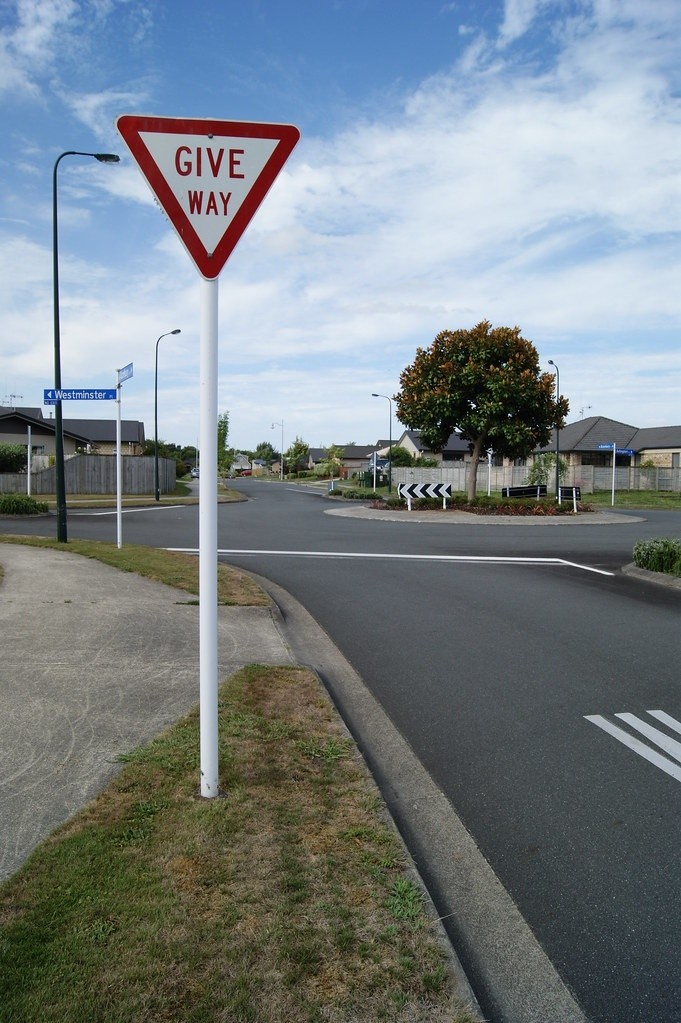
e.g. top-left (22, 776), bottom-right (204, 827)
top-left (190, 468), bottom-right (199, 478)
top-left (241, 470), bottom-right (252, 476)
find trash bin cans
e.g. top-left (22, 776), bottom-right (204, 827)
top-left (356, 471), bottom-right (372, 488)
top-left (372, 474), bottom-right (387, 487)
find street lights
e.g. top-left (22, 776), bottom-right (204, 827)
top-left (53, 148), bottom-right (120, 543)
top-left (154, 328), bottom-right (181, 500)
top-left (548, 358), bottom-right (560, 501)
top-left (372, 393), bottom-right (392, 493)
top-left (271, 422), bottom-right (283, 481)
